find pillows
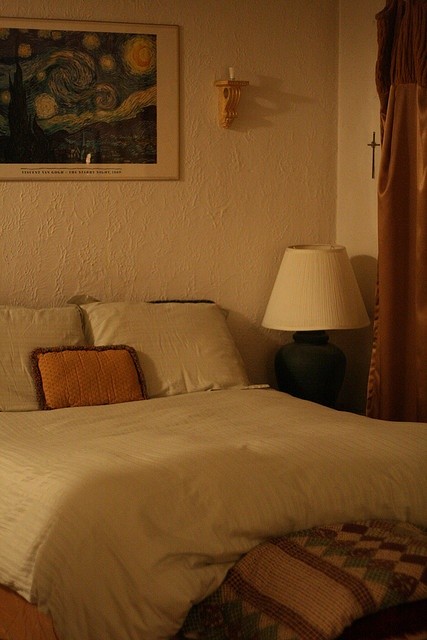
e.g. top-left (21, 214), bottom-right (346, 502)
top-left (81, 301), bottom-right (249, 398)
top-left (0, 302), bottom-right (86, 413)
top-left (29, 343), bottom-right (149, 411)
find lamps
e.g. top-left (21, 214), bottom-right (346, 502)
top-left (261, 243), bottom-right (370, 408)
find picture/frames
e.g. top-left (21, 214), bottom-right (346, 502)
top-left (1, 16), bottom-right (181, 182)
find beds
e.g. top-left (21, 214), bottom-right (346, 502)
top-left (0, 299), bottom-right (427, 640)
